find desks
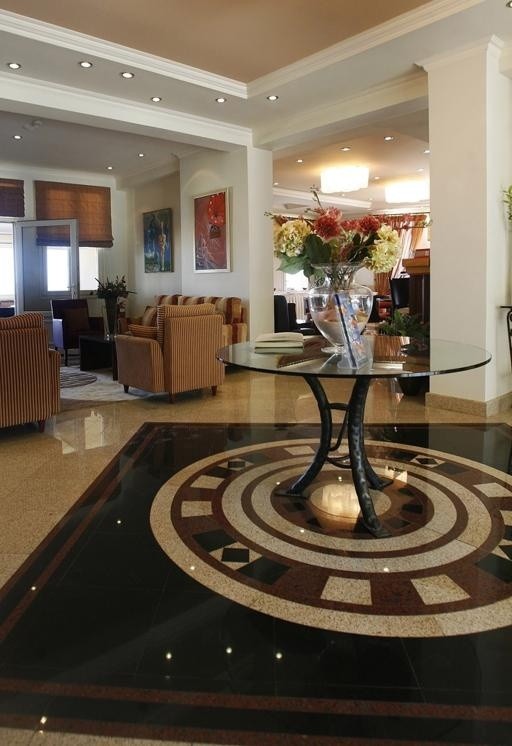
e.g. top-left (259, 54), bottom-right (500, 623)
top-left (214, 333), bottom-right (493, 541)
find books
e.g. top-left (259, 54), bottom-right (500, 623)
top-left (254, 348), bottom-right (303, 354)
top-left (255, 332), bottom-right (303, 346)
top-left (402, 249), bottom-right (430, 274)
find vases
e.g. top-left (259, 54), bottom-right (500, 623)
top-left (304, 262), bottom-right (377, 355)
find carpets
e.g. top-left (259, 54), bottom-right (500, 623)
top-left (58, 365), bottom-right (169, 413)
top-left (59, 372), bottom-right (98, 388)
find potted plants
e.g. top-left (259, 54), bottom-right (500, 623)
top-left (94, 272), bottom-right (139, 338)
top-left (374, 308), bottom-right (429, 396)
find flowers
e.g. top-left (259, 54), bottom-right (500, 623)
top-left (259, 185), bottom-right (404, 299)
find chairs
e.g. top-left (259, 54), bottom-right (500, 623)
top-left (0, 311), bottom-right (62, 435)
top-left (274, 294), bottom-right (323, 335)
top-left (49, 298), bottom-right (104, 368)
top-left (112, 302), bottom-right (225, 404)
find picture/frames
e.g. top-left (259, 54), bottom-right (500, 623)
top-left (142, 206), bottom-right (175, 276)
top-left (191, 186), bottom-right (233, 275)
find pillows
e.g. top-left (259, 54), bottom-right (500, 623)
top-left (141, 303), bottom-right (158, 326)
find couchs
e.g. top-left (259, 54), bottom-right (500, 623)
top-left (117, 293), bottom-right (249, 371)
